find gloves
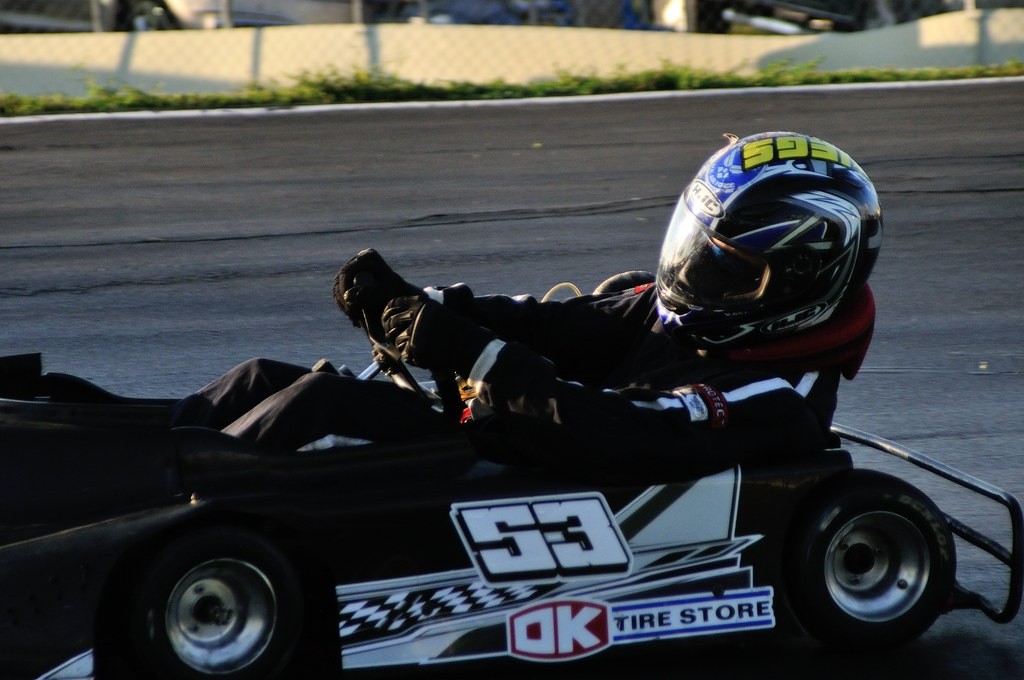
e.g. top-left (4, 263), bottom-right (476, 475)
top-left (382, 296), bottom-right (491, 371)
top-left (333, 248), bottom-right (427, 329)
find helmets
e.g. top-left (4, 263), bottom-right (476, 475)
top-left (655, 132), bottom-right (883, 351)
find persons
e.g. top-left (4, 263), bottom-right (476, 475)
top-left (164, 130), bottom-right (885, 460)
